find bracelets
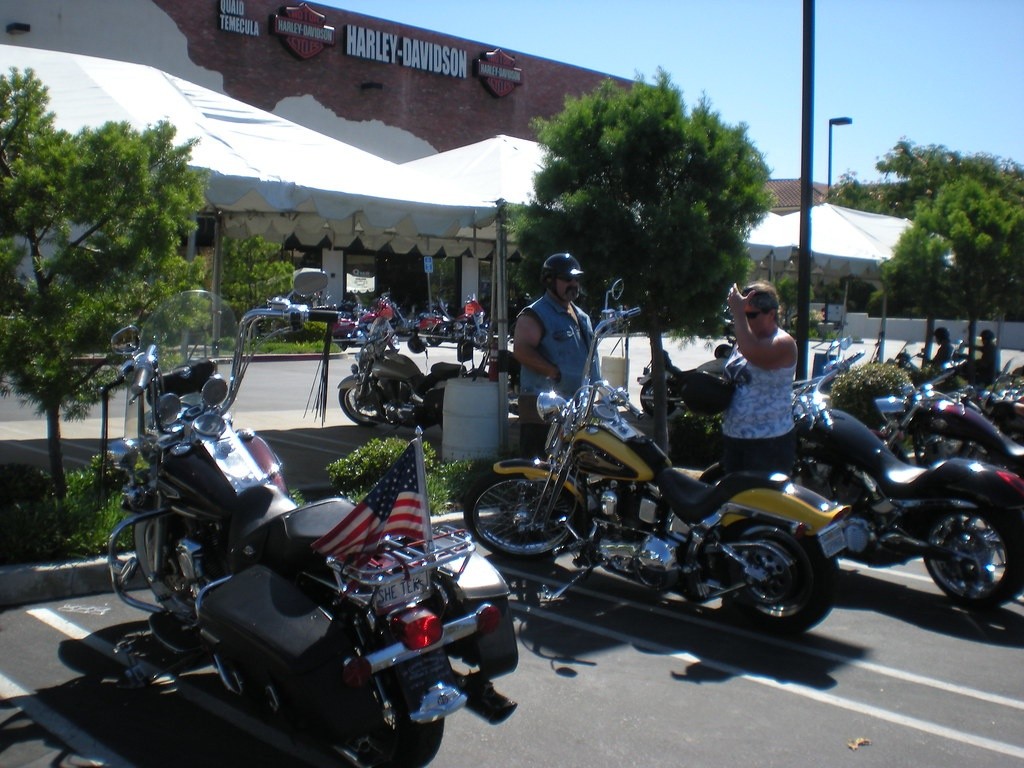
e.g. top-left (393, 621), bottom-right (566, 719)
top-left (553, 371), bottom-right (561, 383)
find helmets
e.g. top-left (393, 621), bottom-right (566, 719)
top-left (682, 371), bottom-right (732, 418)
top-left (542, 253), bottom-right (583, 287)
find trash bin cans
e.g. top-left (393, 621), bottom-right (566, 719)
top-left (598, 355), bottom-right (630, 402)
top-left (812, 352), bottom-right (838, 392)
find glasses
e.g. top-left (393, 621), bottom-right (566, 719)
top-left (744, 310), bottom-right (768, 320)
top-left (553, 275), bottom-right (580, 282)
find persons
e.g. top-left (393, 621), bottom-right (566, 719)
top-left (964, 329), bottom-right (1000, 385)
top-left (721, 278), bottom-right (797, 477)
top-left (917, 327), bottom-right (953, 368)
top-left (508, 252), bottom-right (601, 546)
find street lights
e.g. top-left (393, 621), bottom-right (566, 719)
top-left (821, 115), bottom-right (854, 322)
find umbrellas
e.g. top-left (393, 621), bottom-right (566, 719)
top-left (744, 200), bottom-right (954, 359)
top-left (0, 43), bottom-right (558, 448)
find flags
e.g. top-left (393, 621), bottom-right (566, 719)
top-left (311, 442), bottom-right (424, 597)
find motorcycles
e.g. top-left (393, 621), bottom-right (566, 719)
top-left (328, 287), bottom-right (519, 435)
top-left (104, 290), bottom-right (518, 768)
top-left (462, 277), bottom-right (854, 641)
top-left (635, 319), bottom-right (1024, 613)
